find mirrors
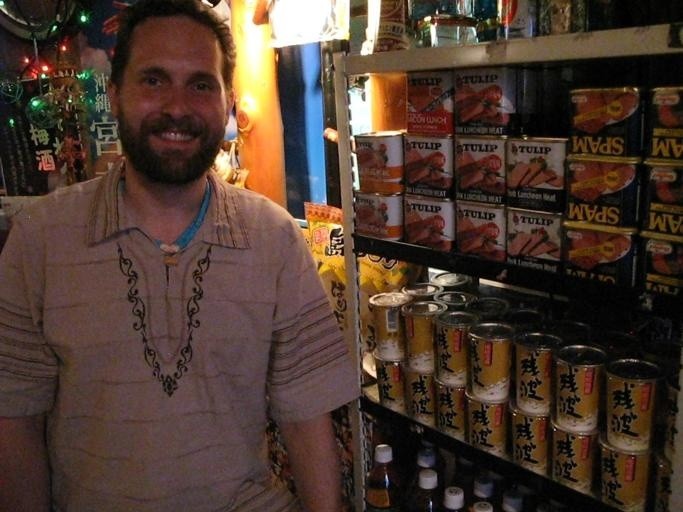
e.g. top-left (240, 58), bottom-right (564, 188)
top-left (274, 39), bottom-right (349, 228)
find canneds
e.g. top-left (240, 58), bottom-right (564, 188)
top-left (354, 135), bottom-right (683, 311)
top-left (371, 272), bottom-right (683, 512)
top-left (566, 86), bottom-right (683, 167)
top-left (370, 0), bottom-right (591, 55)
top-left (402, 68), bottom-right (517, 135)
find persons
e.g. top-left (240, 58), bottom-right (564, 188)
top-left (1, 1), bottom-right (365, 510)
top-left (252, 1), bottom-right (338, 48)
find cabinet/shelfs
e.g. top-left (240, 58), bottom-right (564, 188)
top-left (333, 24), bottom-right (683, 512)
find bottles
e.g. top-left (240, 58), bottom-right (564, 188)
top-left (363, 439), bottom-right (527, 512)
